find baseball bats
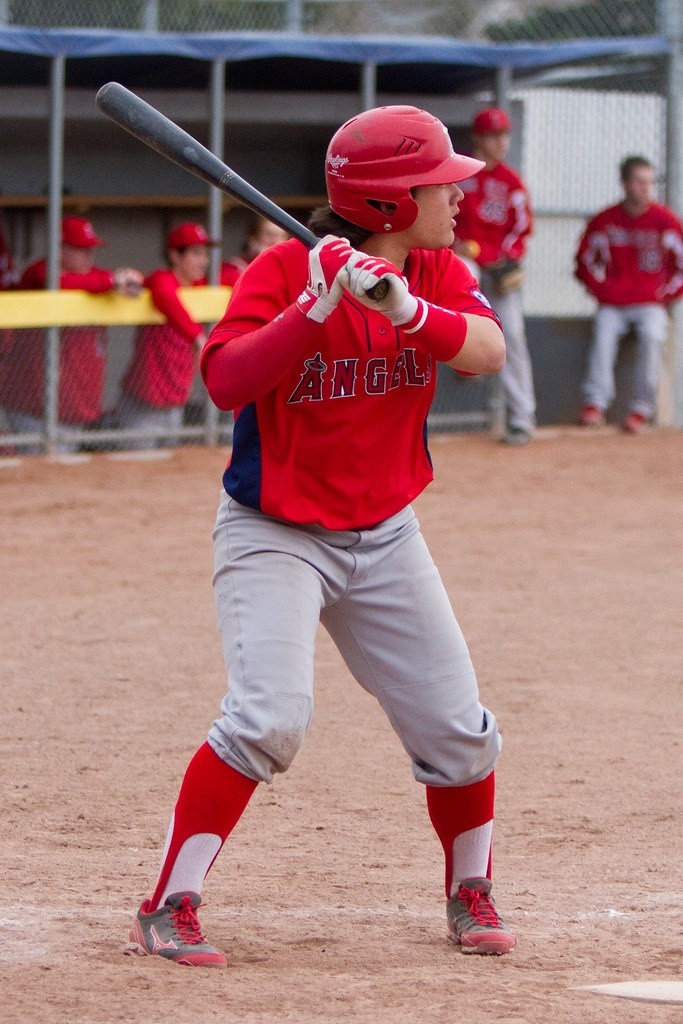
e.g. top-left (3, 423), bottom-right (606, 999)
top-left (97, 82), bottom-right (391, 300)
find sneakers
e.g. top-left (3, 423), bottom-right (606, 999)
top-left (125, 890), bottom-right (227, 969)
top-left (446, 876), bottom-right (517, 955)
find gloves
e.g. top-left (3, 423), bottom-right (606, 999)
top-left (337, 250), bottom-right (419, 327)
top-left (293, 235), bottom-right (355, 324)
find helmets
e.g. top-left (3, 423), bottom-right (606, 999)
top-left (326, 105), bottom-right (486, 234)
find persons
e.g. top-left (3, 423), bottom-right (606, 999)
top-left (0, 216), bottom-right (144, 456)
top-left (209, 212), bottom-right (291, 447)
top-left (127, 104), bottom-right (518, 969)
top-left (453, 109), bottom-right (537, 446)
top-left (575, 157), bottom-right (683, 436)
top-left (111, 223), bottom-right (213, 452)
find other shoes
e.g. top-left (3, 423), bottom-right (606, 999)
top-left (579, 405), bottom-right (603, 426)
top-left (500, 428), bottom-right (530, 444)
top-left (626, 414), bottom-right (646, 431)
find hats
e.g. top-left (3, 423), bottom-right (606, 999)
top-left (475, 109), bottom-right (512, 134)
top-left (168, 224), bottom-right (217, 248)
top-left (62, 217), bottom-right (105, 250)
